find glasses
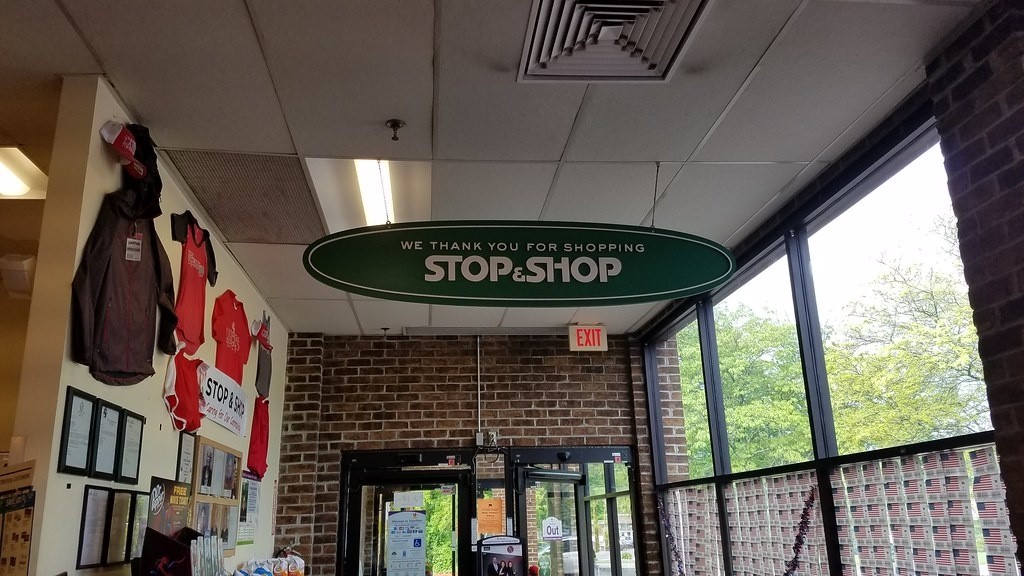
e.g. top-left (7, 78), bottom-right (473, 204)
top-left (207, 454), bottom-right (212, 459)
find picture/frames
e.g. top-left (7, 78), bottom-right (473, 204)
top-left (189, 433), bottom-right (244, 558)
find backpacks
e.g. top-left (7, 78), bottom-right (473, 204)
top-left (119, 123), bottom-right (163, 221)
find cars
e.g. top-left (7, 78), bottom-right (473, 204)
top-left (538, 536), bottom-right (600, 576)
top-left (607, 531), bottom-right (634, 551)
top-left (592, 535), bottom-right (606, 551)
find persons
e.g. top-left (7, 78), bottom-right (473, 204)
top-left (202, 447), bottom-right (214, 486)
top-left (488, 557), bottom-right (517, 576)
top-left (201, 506), bottom-right (228, 542)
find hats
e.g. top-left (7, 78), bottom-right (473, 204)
top-left (99, 120), bottom-right (146, 179)
